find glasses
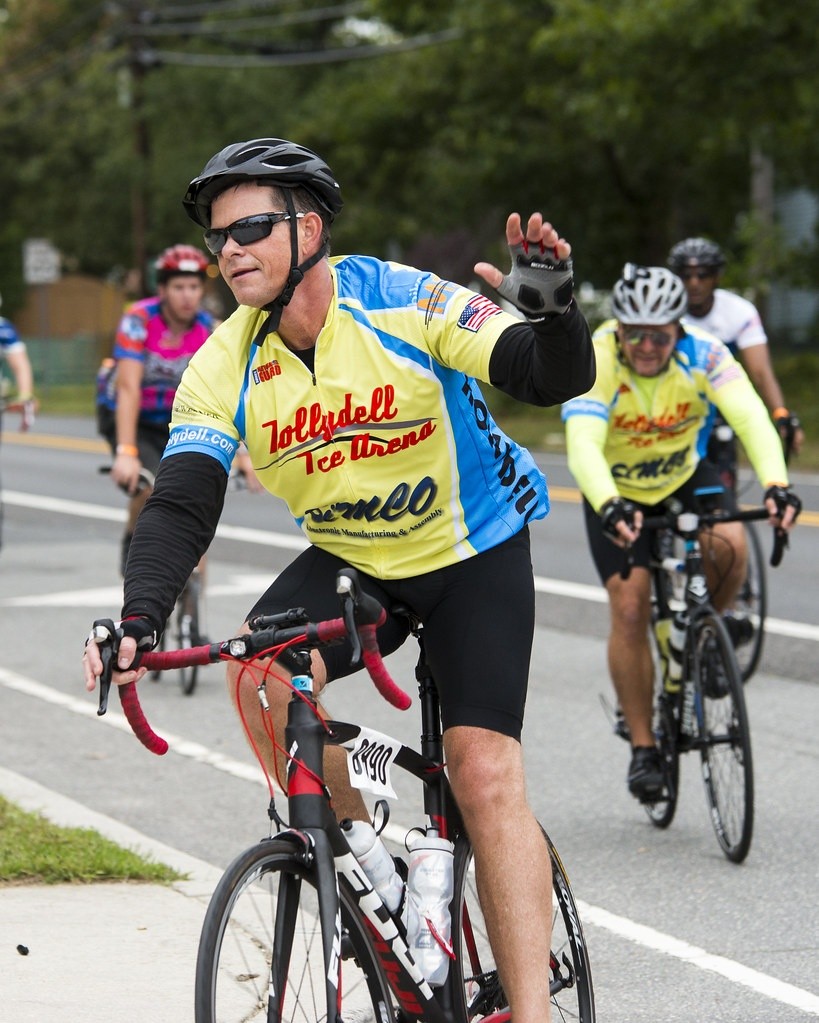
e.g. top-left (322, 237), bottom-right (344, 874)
top-left (619, 326), bottom-right (680, 347)
top-left (681, 269), bottom-right (713, 280)
top-left (202, 210), bottom-right (303, 256)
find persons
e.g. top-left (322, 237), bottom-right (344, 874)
top-left (666, 237), bottom-right (805, 501)
top-left (81, 138), bottom-right (598, 1023)
top-left (0, 315), bottom-right (34, 401)
top-left (111, 245), bottom-right (264, 646)
top-left (559, 267), bottom-right (802, 797)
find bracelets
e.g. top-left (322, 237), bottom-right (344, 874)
top-left (773, 407), bottom-right (788, 419)
top-left (116, 444), bottom-right (138, 457)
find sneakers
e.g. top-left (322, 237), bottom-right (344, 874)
top-left (699, 635), bottom-right (729, 701)
top-left (628, 744), bottom-right (667, 797)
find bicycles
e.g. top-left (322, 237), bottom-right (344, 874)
top-left (92, 568), bottom-right (596, 1022)
top-left (612, 414), bottom-right (800, 864)
top-left (93, 458), bottom-right (249, 696)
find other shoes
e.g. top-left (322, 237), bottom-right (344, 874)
top-left (122, 530), bottom-right (134, 576)
top-left (341, 858), bottom-right (407, 958)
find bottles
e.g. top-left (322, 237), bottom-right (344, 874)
top-left (668, 610), bottom-right (689, 684)
top-left (404, 825), bottom-right (456, 987)
top-left (653, 601), bottom-right (684, 695)
top-left (339, 818), bottom-right (404, 914)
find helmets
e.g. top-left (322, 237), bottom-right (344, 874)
top-left (610, 261), bottom-right (689, 326)
top-left (666, 236), bottom-right (726, 273)
top-left (180, 136), bottom-right (343, 229)
top-left (153, 244), bottom-right (209, 282)
